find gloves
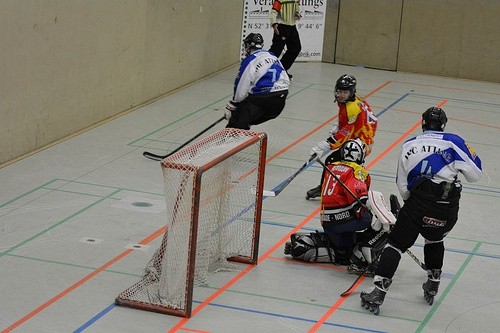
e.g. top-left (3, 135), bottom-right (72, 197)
top-left (225, 100), bottom-right (238, 120)
top-left (329, 123), bottom-right (339, 134)
top-left (309, 140), bottom-right (332, 159)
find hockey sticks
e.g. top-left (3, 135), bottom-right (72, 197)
top-left (250, 129), bottom-right (336, 196)
top-left (340, 249), bottom-right (384, 297)
top-left (142, 115), bottom-right (227, 162)
top-left (316, 157), bottom-right (426, 271)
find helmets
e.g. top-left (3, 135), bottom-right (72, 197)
top-left (422, 106), bottom-right (448, 131)
top-left (340, 138), bottom-right (367, 162)
top-left (333, 74), bottom-right (357, 104)
top-left (242, 33), bottom-right (265, 49)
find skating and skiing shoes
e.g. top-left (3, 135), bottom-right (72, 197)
top-left (284, 232), bottom-right (299, 256)
top-left (422, 275), bottom-right (440, 305)
top-left (347, 256), bottom-right (376, 277)
top-left (360, 286), bottom-right (386, 315)
top-left (306, 186), bottom-right (322, 200)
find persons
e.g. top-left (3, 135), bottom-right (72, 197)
top-left (290, 139), bottom-right (401, 278)
top-left (268, 0), bottom-right (302, 78)
top-left (223, 32), bottom-right (290, 130)
top-left (360, 106), bottom-right (482, 305)
top-left (306, 74), bottom-right (378, 200)
